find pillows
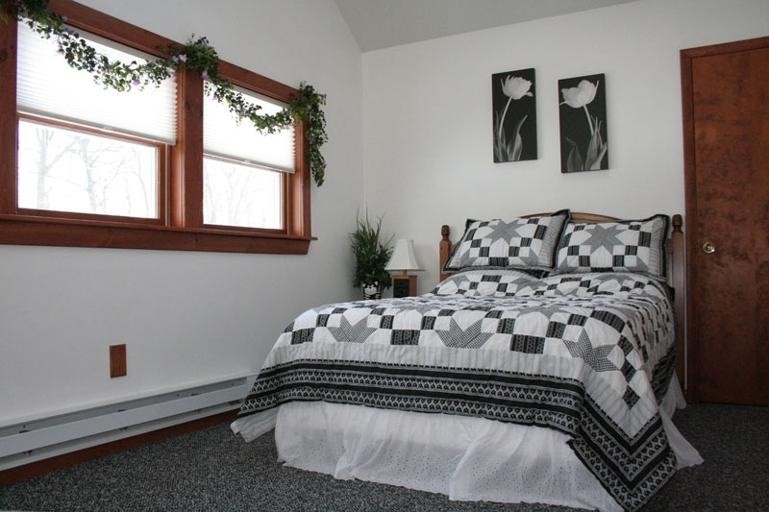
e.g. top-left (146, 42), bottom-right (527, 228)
top-left (442, 209), bottom-right (571, 272)
top-left (540, 213), bottom-right (671, 280)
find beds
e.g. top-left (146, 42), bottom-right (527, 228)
top-left (229, 213), bottom-right (704, 512)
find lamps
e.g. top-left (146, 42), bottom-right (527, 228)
top-left (383, 239), bottom-right (425, 297)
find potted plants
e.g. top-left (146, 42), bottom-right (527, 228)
top-left (348, 205), bottom-right (392, 301)
